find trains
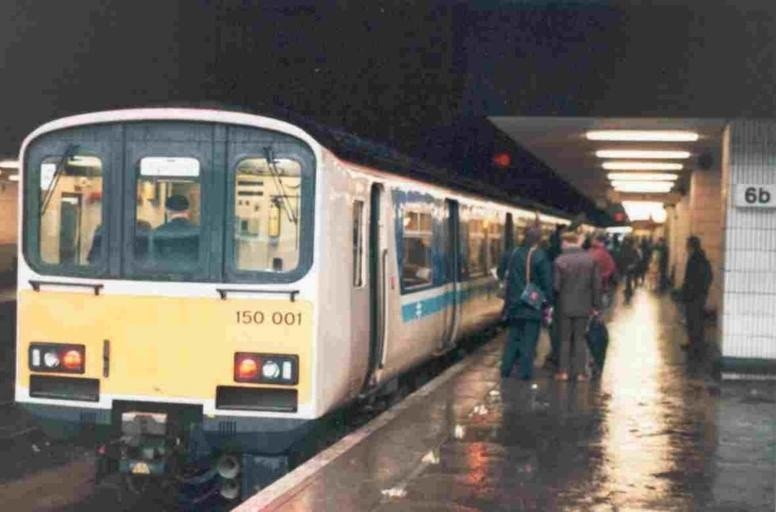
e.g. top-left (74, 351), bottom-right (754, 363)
top-left (18, 108), bottom-right (603, 506)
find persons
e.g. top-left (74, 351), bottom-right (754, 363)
top-left (496, 223), bottom-right (669, 382)
top-left (677, 234), bottom-right (712, 356)
top-left (85, 220), bottom-right (153, 266)
top-left (147, 194), bottom-right (197, 269)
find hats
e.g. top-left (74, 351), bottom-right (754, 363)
top-left (561, 233), bottom-right (578, 241)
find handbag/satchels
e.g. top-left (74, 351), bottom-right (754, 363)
top-left (521, 285), bottom-right (549, 311)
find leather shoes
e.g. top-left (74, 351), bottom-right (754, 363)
top-left (551, 371), bottom-right (591, 383)
